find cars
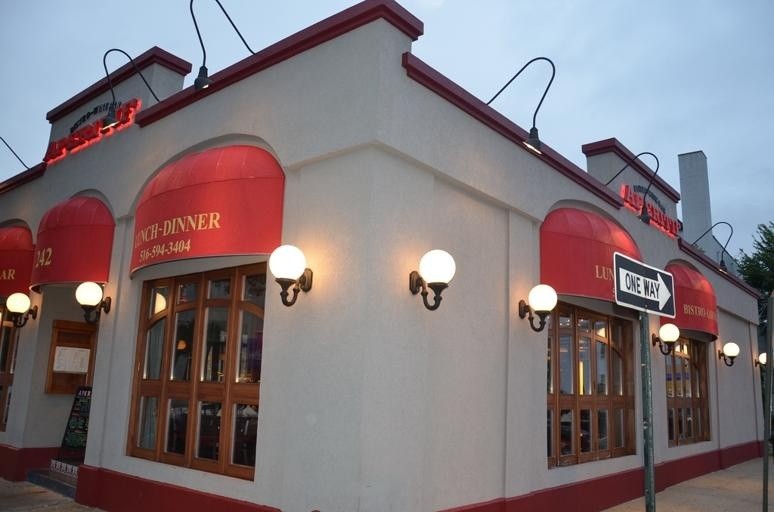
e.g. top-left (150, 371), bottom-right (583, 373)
top-left (546, 410), bottom-right (608, 462)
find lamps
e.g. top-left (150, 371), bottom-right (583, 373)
top-left (189, 0), bottom-right (255, 91)
top-left (652, 323), bottom-right (680, 355)
top-left (691, 222), bottom-right (733, 274)
top-left (518, 284), bottom-right (558, 332)
top-left (487, 57), bottom-right (555, 154)
top-left (102, 49), bottom-right (159, 130)
top-left (269, 245), bottom-right (313, 306)
top-left (717, 341), bottom-right (739, 366)
top-left (76, 281), bottom-right (111, 323)
top-left (755, 353), bottom-right (767, 367)
top-left (6, 293), bottom-right (37, 328)
top-left (605, 152), bottom-right (660, 224)
top-left (408, 249), bottom-right (455, 310)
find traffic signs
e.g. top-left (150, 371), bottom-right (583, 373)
top-left (614, 251), bottom-right (677, 318)
